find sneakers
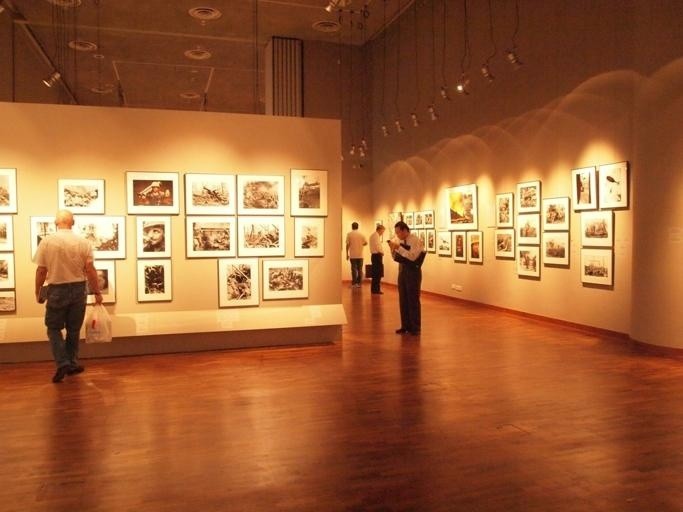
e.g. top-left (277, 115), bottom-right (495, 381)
top-left (352, 285), bottom-right (361, 288)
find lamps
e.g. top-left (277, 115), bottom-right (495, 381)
top-left (337, 0), bottom-right (523, 162)
top-left (322, 0), bottom-right (338, 13)
top-left (43, 71), bottom-right (61, 89)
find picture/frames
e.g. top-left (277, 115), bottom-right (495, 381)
top-left (374, 161), bottom-right (630, 287)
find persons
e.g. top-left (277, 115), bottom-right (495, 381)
top-left (143, 222), bottom-right (164, 252)
top-left (369, 225), bottom-right (386, 294)
top-left (138, 182), bottom-right (170, 206)
top-left (32, 209), bottom-right (103, 383)
top-left (389, 221), bottom-right (424, 336)
top-left (346, 222), bottom-right (368, 288)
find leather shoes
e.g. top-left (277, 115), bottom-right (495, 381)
top-left (409, 328), bottom-right (420, 334)
top-left (68, 365), bottom-right (84, 375)
top-left (396, 328), bottom-right (406, 334)
top-left (378, 292), bottom-right (383, 295)
top-left (52, 365), bottom-right (70, 383)
